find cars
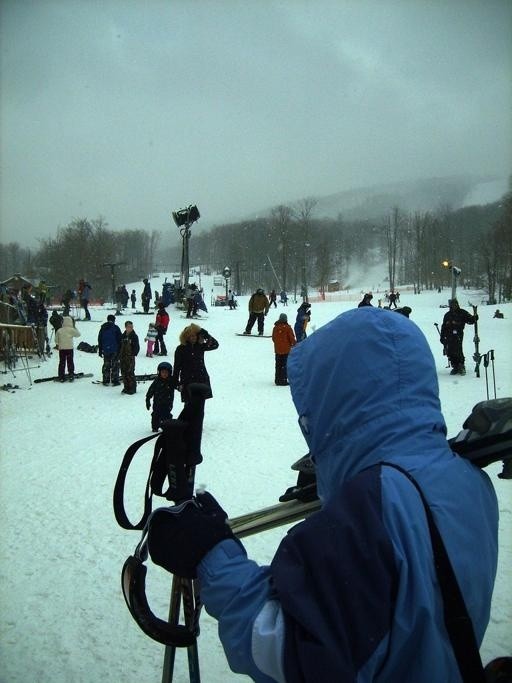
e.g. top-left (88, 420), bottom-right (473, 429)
top-left (152, 266), bottom-right (212, 277)
top-left (213, 274), bottom-right (222, 285)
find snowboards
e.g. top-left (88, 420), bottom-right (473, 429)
top-left (236, 334), bottom-right (273, 337)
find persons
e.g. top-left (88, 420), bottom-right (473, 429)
top-left (242, 288), bottom-right (311, 386)
top-left (173, 323), bottom-right (221, 403)
top-left (141, 278), bottom-right (237, 358)
top-left (358, 292), bottom-right (478, 376)
top-left (33, 278), bottom-right (140, 394)
top-left (146, 304), bottom-right (497, 682)
top-left (145, 361), bottom-right (180, 431)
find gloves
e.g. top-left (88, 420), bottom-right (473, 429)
top-left (170, 488), bottom-right (235, 568)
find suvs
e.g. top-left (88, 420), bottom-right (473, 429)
top-left (211, 286), bottom-right (230, 305)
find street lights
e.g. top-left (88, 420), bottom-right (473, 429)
top-left (172, 202), bottom-right (200, 287)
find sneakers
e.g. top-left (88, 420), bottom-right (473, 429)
top-left (83, 317), bottom-right (91, 321)
top-left (116, 312), bottom-right (122, 315)
top-left (60, 378), bottom-right (67, 382)
top-left (186, 313), bottom-right (201, 318)
top-left (152, 351), bottom-right (159, 354)
top-left (69, 377), bottom-right (74, 382)
top-left (259, 333), bottom-right (263, 336)
top-left (450, 368), bottom-right (466, 375)
top-left (275, 382), bottom-right (290, 386)
top-left (103, 382), bottom-right (110, 386)
top-left (112, 382), bottom-right (121, 386)
top-left (122, 388), bottom-right (137, 395)
top-left (157, 352), bottom-right (167, 356)
top-left (243, 331), bottom-right (252, 334)
top-left (146, 354), bottom-right (152, 357)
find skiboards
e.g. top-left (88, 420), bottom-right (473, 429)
top-left (34, 373), bottom-right (93, 383)
top-left (225, 396), bottom-right (512, 541)
top-left (92, 374), bottom-right (159, 385)
top-left (473, 305), bottom-right (480, 377)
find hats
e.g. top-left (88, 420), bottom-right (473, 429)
top-left (279, 313), bottom-right (287, 323)
